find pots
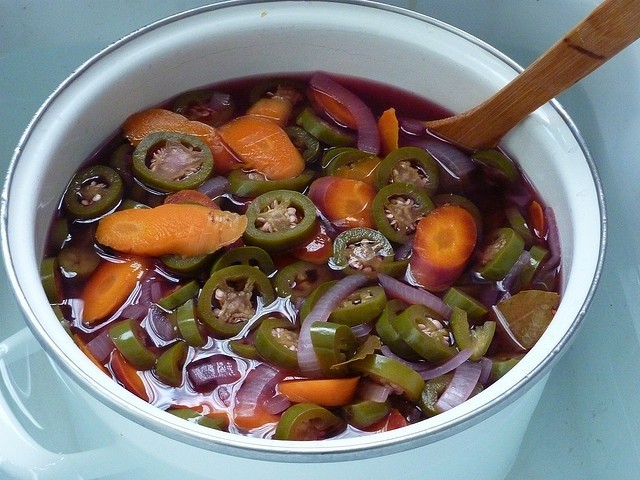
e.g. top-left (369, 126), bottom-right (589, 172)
top-left (0, 0), bottom-right (608, 479)
